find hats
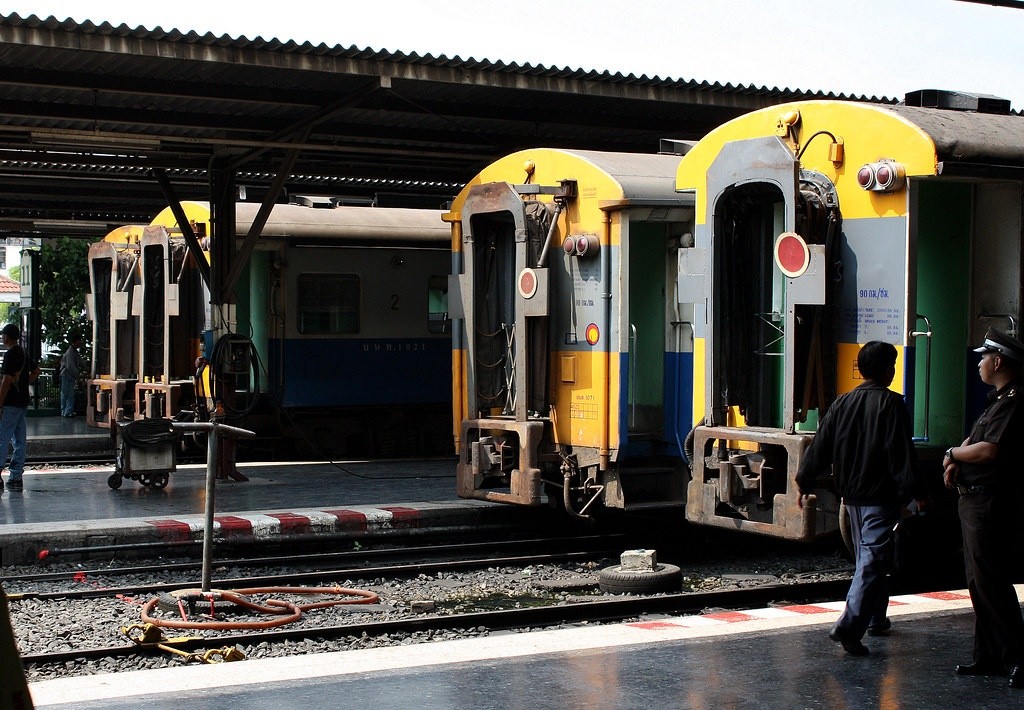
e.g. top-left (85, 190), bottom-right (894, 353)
top-left (973, 326), bottom-right (1024, 360)
top-left (0, 324), bottom-right (20, 338)
top-left (66, 327), bottom-right (84, 339)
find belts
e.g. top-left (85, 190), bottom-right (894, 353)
top-left (955, 480), bottom-right (1012, 498)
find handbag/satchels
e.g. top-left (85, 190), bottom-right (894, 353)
top-left (51, 367), bottom-right (61, 384)
top-left (0, 374), bottom-right (16, 398)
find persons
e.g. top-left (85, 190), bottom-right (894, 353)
top-left (60, 335), bottom-right (91, 418)
top-left (943, 326), bottom-right (1024, 689)
top-left (795, 342), bottom-right (924, 656)
top-left (0, 324), bottom-right (41, 490)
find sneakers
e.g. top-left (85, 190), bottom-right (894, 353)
top-left (0, 478), bottom-right (4, 489)
top-left (5, 478), bottom-right (23, 488)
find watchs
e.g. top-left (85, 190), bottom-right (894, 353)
top-left (946, 447), bottom-right (953, 460)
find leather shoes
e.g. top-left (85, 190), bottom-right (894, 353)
top-left (867, 618), bottom-right (892, 636)
top-left (956, 658), bottom-right (1006, 676)
top-left (828, 624), bottom-right (870, 657)
top-left (1006, 664), bottom-right (1024, 688)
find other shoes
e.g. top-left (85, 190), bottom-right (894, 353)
top-left (60, 412), bottom-right (77, 418)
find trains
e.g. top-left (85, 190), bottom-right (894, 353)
top-left (674, 97), bottom-right (1024, 577)
top-left (83, 224), bottom-right (145, 432)
top-left (120, 195), bottom-right (452, 465)
top-left (440, 139), bottom-right (695, 519)
